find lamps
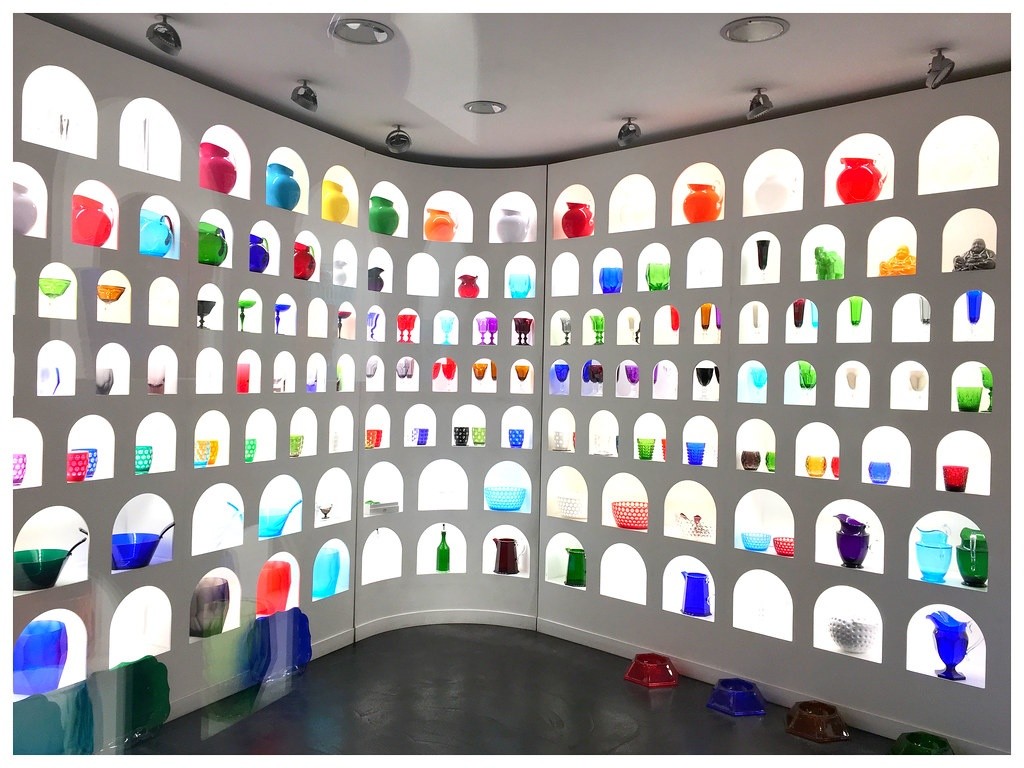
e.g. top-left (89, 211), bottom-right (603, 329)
top-left (618, 117), bottom-right (641, 146)
top-left (291, 80), bottom-right (318, 111)
top-left (747, 88), bottom-right (774, 120)
top-left (926, 47), bottom-right (955, 90)
top-left (386, 124), bottom-right (411, 154)
top-left (146, 15), bottom-right (181, 55)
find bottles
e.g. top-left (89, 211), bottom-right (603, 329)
top-left (14, 142), bottom-right (885, 300)
top-left (436, 523), bottom-right (450, 573)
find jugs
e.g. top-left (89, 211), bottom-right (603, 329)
top-left (834, 514), bottom-right (869, 570)
top-left (915, 526), bottom-right (988, 589)
top-left (492, 538), bottom-right (713, 618)
top-left (927, 610), bottom-right (985, 681)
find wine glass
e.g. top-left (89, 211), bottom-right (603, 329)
top-left (318, 504), bottom-right (334, 522)
top-left (34, 277), bottom-right (993, 412)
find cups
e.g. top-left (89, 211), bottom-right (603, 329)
top-left (599, 267), bottom-right (623, 294)
top-left (147, 364), bottom-right (165, 396)
top-left (958, 386), bottom-right (983, 412)
top-left (189, 548), bottom-right (340, 637)
top-left (645, 263), bottom-right (672, 292)
top-left (95, 369), bottom-right (115, 395)
top-left (509, 273), bottom-right (532, 299)
top-left (11, 428), bottom-right (969, 491)
top-left (235, 355), bottom-right (250, 395)
top-left (13, 621), bottom-right (68, 695)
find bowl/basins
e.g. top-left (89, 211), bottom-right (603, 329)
top-left (553, 494), bottom-right (586, 518)
top-left (227, 499), bottom-right (302, 537)
top-left (111, 534), bottom-right (159, 571)
top-left (484, 486), bottom-right (528, 512)
top-left (13, 520), bottom-right (87, 591)
top-left (612, 501), bottom-right (648, 531)
top-left (742, 531), bottom-right (795, 558)
